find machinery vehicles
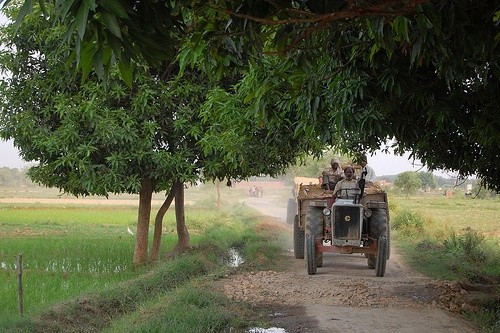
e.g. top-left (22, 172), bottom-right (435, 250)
top-left (288, 165), bottom-right (390, 277)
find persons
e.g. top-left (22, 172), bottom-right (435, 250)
top-left (334, 167), bottom-right (358, 199)
top-left (321, 158), bottom-right (345, 191)
top-left (253, 186), bottom-right (256, 193)
top-left (358, 159), bottom-right (375, 186)
